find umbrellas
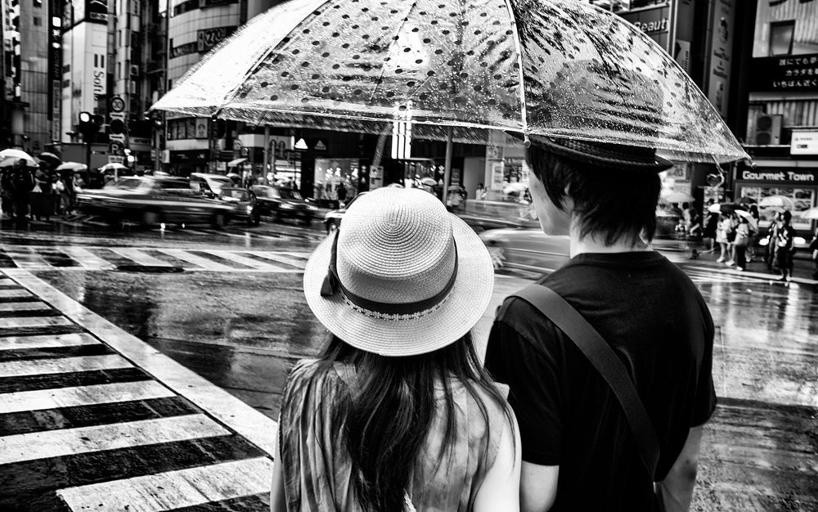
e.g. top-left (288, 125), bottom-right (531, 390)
top-left (148, 0), bottom-right (754, 212)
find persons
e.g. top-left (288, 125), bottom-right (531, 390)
top-left (268, 183), bottom-right (523, 511)
top-left (484, 139), bottom-right (712, 511)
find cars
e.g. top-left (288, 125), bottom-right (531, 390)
top-left (504, 182), bottom-right (522, 196)
top-left (454, 199), bottom-right (572, 277)
top-left (75, 171), bottom-right (317, 232)
top-left (654, 207), bottom-right (678, 235)
top-left (323, 191), bottom-right (371, 233)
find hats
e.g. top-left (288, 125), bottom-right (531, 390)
top-left (303, 185), bottom-right (496, 357)
top-left (504, 58), bottom-right (675, 175)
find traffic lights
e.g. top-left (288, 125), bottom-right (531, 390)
top-left (80, 112), bottom-right (90, 135)
top-left (95, 116), bottom-right (105, 141)
top-left (159, 135), bottom-right (164, 149)
